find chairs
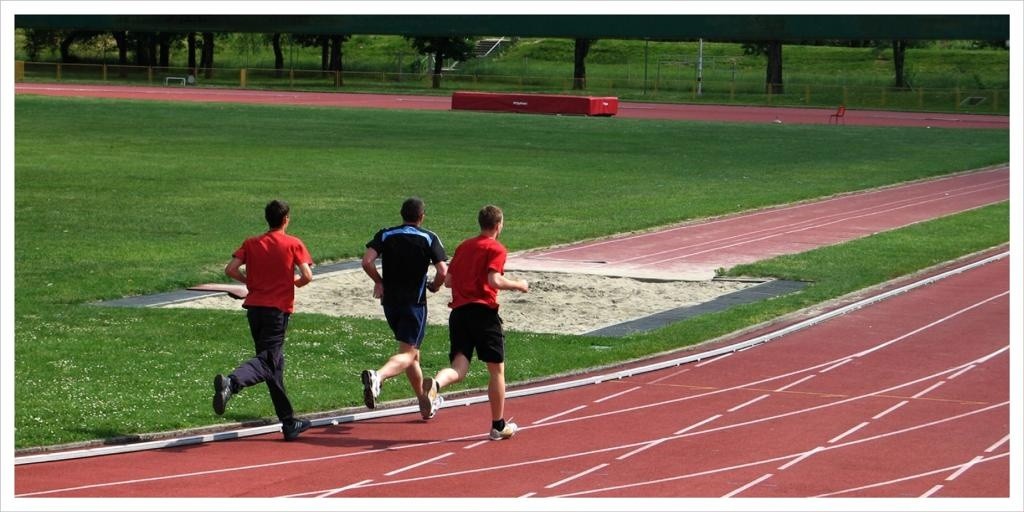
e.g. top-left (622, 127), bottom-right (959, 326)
top-left (827, 106), bottom-right (847, 128)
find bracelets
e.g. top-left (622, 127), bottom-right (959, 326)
top-left (430, 282), bottom-right (440, 291)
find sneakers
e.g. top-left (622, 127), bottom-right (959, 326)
top-left (424, 394), bottom-right (444, 420)
top-left (282, 417), bottom-right (312, 442)
top-left (361, 368), bottom-right (383, 411)
top-left (417, 375), bottom-right (439, 419)
top-left (212, 372), bottom-right (234, 417)
top-left (488, 420), bottom-right (518, 442)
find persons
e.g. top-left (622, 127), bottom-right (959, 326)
top-left (359, 196), bottom-right (449, 421)
top-left (418, 204), bottom-right (531, 441)
top-left (210, 199), bottom-right (315, 440)
